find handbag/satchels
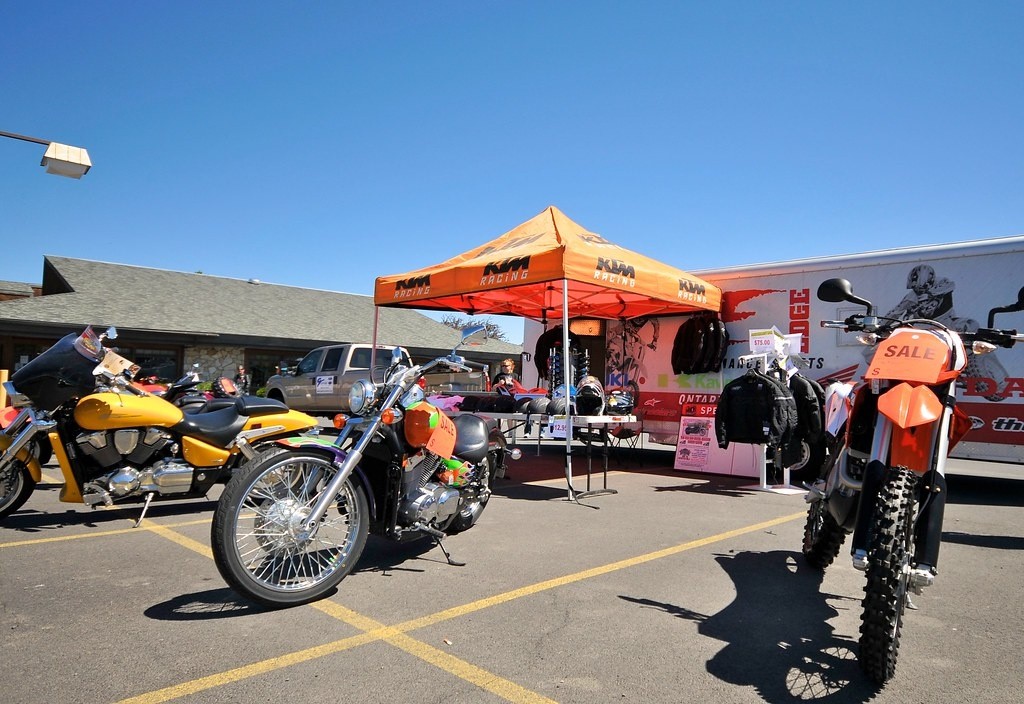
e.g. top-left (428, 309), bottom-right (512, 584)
top-left (453, 393), bottom-right (518, 415)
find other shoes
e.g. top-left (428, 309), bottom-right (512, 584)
top-left (523, 433), bottom-right (529, 438)
top-left (540, 434), bottom-right (545, 438)
top-left (508, 431), bottom-right (513, 438)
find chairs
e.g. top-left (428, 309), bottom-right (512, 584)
top-left (607, 424), bottom-right (644, 458)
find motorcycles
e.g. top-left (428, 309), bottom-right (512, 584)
top-left (0, 326), bottom-right (324, 529)
top-left (802, 279), bottom-right (1024, 683)
top-left (211, 325), bottom-right (521, 607)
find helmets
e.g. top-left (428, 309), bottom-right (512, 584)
top-left (520, 375), bottom-right (605, 416)
top-left (606, 388), bottom-right (634, 416)
top-left (432, 455), bottom-right (475, 488)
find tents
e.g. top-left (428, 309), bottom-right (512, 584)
top-left (372, 206), bottom-right (724, 501)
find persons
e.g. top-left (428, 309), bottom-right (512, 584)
top-left (233, 364), bottom-right (250, 397)
top-left (491, 358), bottom-right (522, 392)
top-left (274, 365), bottom-right (280, 375)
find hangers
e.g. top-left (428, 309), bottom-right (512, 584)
top-left (743, 363), bottom-right (762, 377)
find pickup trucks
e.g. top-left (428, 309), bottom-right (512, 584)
top-left (266, 344), bottom-right (490, 413)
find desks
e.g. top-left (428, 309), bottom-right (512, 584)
top-left (443, 410), bottom-right (638, 501)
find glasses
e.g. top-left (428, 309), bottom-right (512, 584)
top-left (502, 365), bottom-right (510, 367)
